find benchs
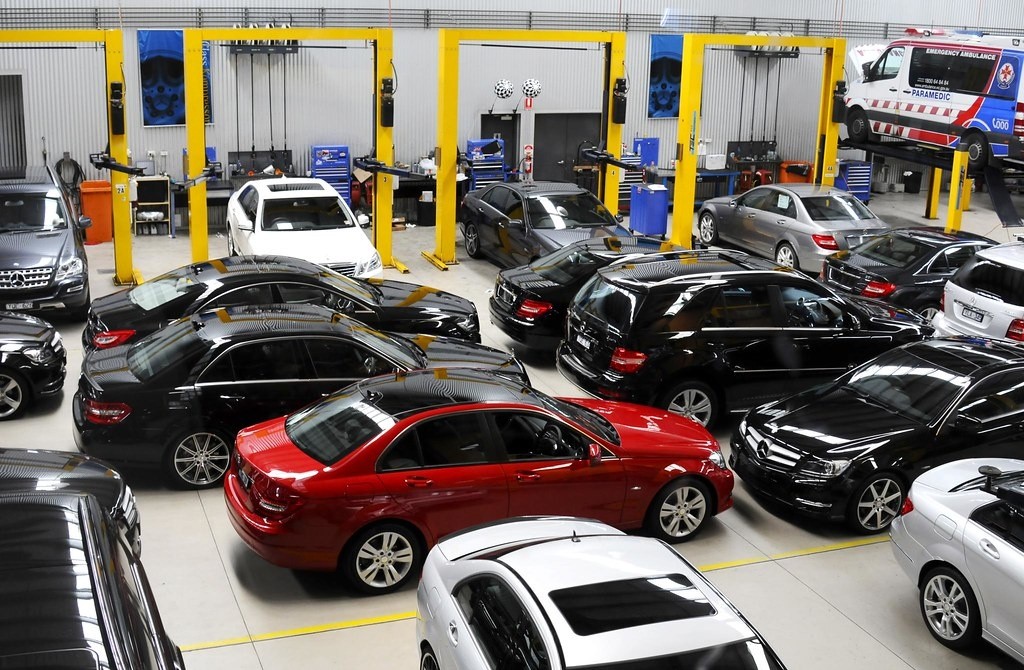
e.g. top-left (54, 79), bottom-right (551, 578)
top-left (879, 245), bottom-right (917, 264)
top-left (334, 407), bottom-right (372, 445)
top-left (792, 206), bottom-right (840, 219)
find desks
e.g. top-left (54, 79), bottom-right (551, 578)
top-left (657, 168), bottom-right (742, 211)
top-left (169, 179), bottom-right (234, 240)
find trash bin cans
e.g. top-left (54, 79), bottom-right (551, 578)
top-left (79, 180), bottom-right (111, 242)
top-left (903, 169), bottom-right (923, 193)
top-left (780, 160), bottom-right (811, 183)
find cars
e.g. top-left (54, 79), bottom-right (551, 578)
top-left (456, 179), bottom-right (632, 272)
top-left (221, 368), bottom-right (740, 594)
top-left (67, 304), bottom-right (538, 495)
top-left (225, 172), bottom-right (380, 284)
top-left (416, 513), bottom-right (791, 670)
top-left (0, 442), bottom-right (145, 562)
top-left (932, 240), bottom-right (1024, 356)
top-left (891, 455), bottom-right (1024, 670)
top-left (0, 177), bottom-right (90, 317)
top-left (696, 182), bottom-right (892, 275)
top-left (728, 334), bottom-right (1024, 536)
top-left (0, 309), bottom-right (70, 421)
top-left (490, 232), bottom-right (697, 350)
top-left (74, 252), bottom-right (483, 356)
top-left (822, 226), bottom-right (1003, 326)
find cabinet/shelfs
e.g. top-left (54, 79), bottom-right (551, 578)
top-left (131, 172), bottom-right (172, 241)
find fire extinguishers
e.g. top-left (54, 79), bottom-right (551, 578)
top-left (519, 152), bottom-right (533, 174)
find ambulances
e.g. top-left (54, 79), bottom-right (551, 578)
top-left (836, 28), bottom-right (1024, 163)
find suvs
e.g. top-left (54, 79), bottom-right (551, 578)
top-left (557, 250), bottom-right (935, 440)
top-left (0, 493), bottom-right (187, 670)
